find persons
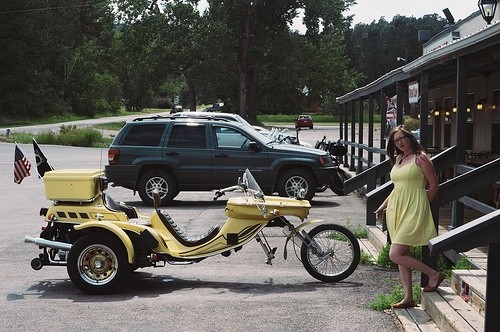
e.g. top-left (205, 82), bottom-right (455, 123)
top-left (373, 124), bottom-right (446, 309)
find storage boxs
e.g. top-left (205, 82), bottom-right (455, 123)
top-left (41, 167), bottom-right (105, 206)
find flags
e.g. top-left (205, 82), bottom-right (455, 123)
top-left (13, 143), bottom-right (31, 185)
top-left (32, 137), bottom-right (53, 179)
top-left (384, 94), bottom-right (397, 125)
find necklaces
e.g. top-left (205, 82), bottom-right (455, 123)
top-left (399, 152), bottom-right (411, 165)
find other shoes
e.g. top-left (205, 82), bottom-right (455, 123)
top-left (423, 271), bottom-right (445, 292)
top-left (392, 299), bottom-right (415, 308)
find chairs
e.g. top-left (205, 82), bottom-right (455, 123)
top-left (152, 187), bottom-right (222, 249)
top-left (99, 174), bottom-right (139, 219)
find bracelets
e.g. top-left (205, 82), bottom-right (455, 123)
top-left (382, 202), bottom-right (388, 207)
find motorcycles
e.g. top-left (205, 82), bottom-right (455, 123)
top-left (23, 168), bottom-right (361, 295)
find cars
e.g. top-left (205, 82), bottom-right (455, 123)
top-left (207, 102), bottom-right (224, 112)
top-left (294, 115), bottom-right (314, 130)
top-left (170, 105), bottom-right (183, 114)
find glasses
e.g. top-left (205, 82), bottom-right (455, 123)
top-left (393, 135), bottom-right (407, 146)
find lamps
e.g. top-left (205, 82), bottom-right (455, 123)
top-left (397, 57), bottom-right (406, 66)
top-left (450, 30), bottom-right (460, 41)
top-left (476, 96), bottom-right (487, 112)
top-left (476, 0), bottom-right (500, 25)
top-left (452, 102), bottom-right (457, 115)
top-left (434, 106), bottom-right (442, 118)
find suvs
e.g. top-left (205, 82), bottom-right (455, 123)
top-left (102, 112), bottom-right (338, 204)
